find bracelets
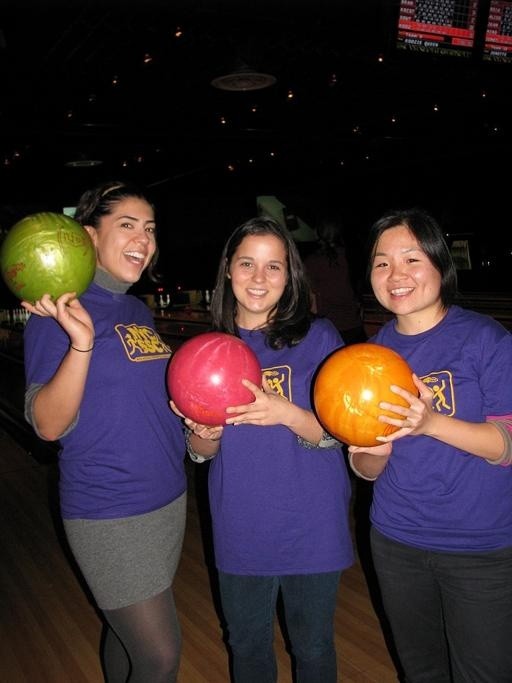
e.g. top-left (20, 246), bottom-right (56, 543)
top-left (70, 344), bottom-right (95, 354)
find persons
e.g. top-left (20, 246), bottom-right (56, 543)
top-left (169, 216), bottom-right (355, 683)
top-left (23, 181), bottom-right (187, 683)
top-left (343, 209), bottom-right (512, 683)
